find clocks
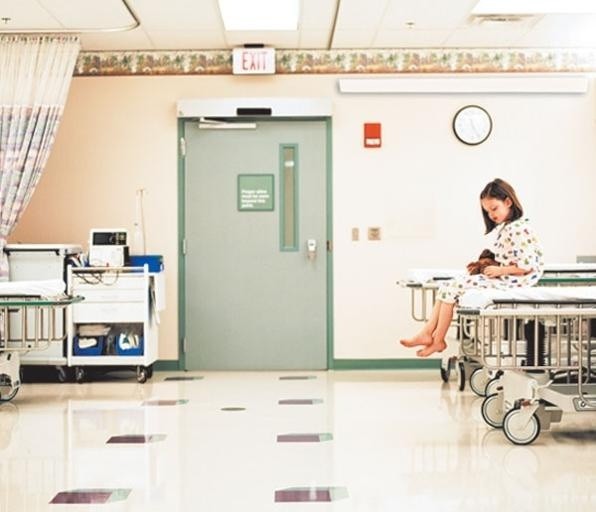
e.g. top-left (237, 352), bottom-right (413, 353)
top-left (452, 104), bottom-right (493, 145)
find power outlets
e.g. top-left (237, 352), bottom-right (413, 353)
top-left (368, 227), bottom-right (382, 241)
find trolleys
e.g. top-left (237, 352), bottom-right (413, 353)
top-left (405, 263), bottom-right (595, 446)
top-left (0, 228), bottom-right (167, 383)
top-left (0, 280), bottom-right (85, 401)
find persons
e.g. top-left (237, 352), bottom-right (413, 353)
top-left (398, 177), bottom-right (544, 359)
